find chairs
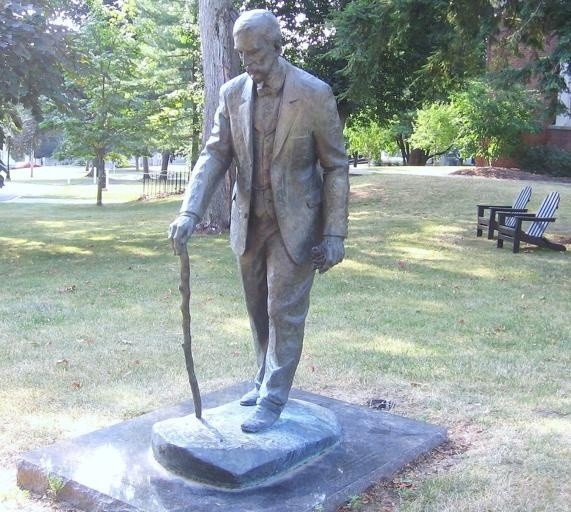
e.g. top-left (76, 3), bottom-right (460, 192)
top-left (476, 185), bottom-right (566, 254)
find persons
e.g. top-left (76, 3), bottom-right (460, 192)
top-left (165, 8), bottom-right (350, 433)
